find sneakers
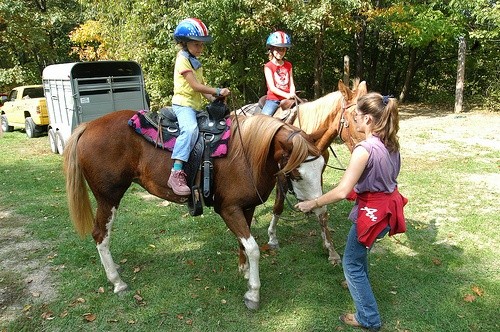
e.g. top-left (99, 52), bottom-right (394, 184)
top-left (167, 168), bottom-right (191, 195)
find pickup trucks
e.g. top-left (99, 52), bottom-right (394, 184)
top-left (0, 84), bottom-right (45, 138)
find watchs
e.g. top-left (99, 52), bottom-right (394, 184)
top-left (216, 88), bottom-right (220, 96)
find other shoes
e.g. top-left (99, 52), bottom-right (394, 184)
top-left (339, 313), bottom-right (360, 326)
top-left (341, 280), bottom-right (348, 288)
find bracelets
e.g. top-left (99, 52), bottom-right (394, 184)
top-left (315, 197), bottom-right (319, 207)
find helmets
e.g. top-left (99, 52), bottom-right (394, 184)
top-left (174, 18), bottom-right (212, 42)
top-left (266, 31), bottom-right (292, 51)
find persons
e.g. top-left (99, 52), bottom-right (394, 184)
top-left (170, 18), bottom-right (229, 195)
top-left (295, 94), bottom-right (407, 327)
top-left (260, 30), bottom-right (295, 117)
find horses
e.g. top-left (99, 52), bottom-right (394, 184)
top-left (230, 76), bottom-right (368, 266)
top-left (62, 110), bottom-right (329, 312)
